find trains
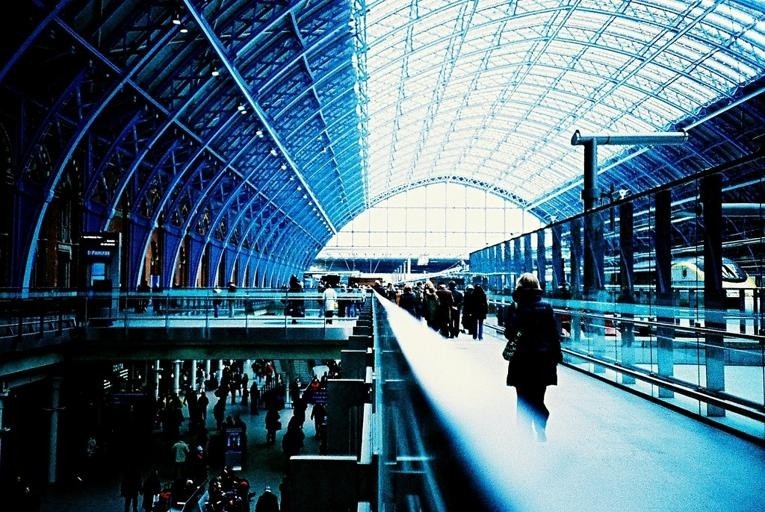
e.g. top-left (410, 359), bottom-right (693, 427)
top-left (528, 256), bottom-right (762, 316)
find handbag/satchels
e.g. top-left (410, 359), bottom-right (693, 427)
top-left (503, 330), bottom-right (523, 361)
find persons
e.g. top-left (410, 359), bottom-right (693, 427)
top-left (212, 281), bottom-right (236, 317)
top-left (114, 359), bottom-right (343, 512)
top-left (282, 275), bottom-right (306, 324)
top-left (373, 278), bottom-right (489, 341)
top-left (502, 272), bottom-right (564, 443)
top-left (136, 277), bottom-right (181, 314)
top-left (317, 278), bottom-right (372, 324)
